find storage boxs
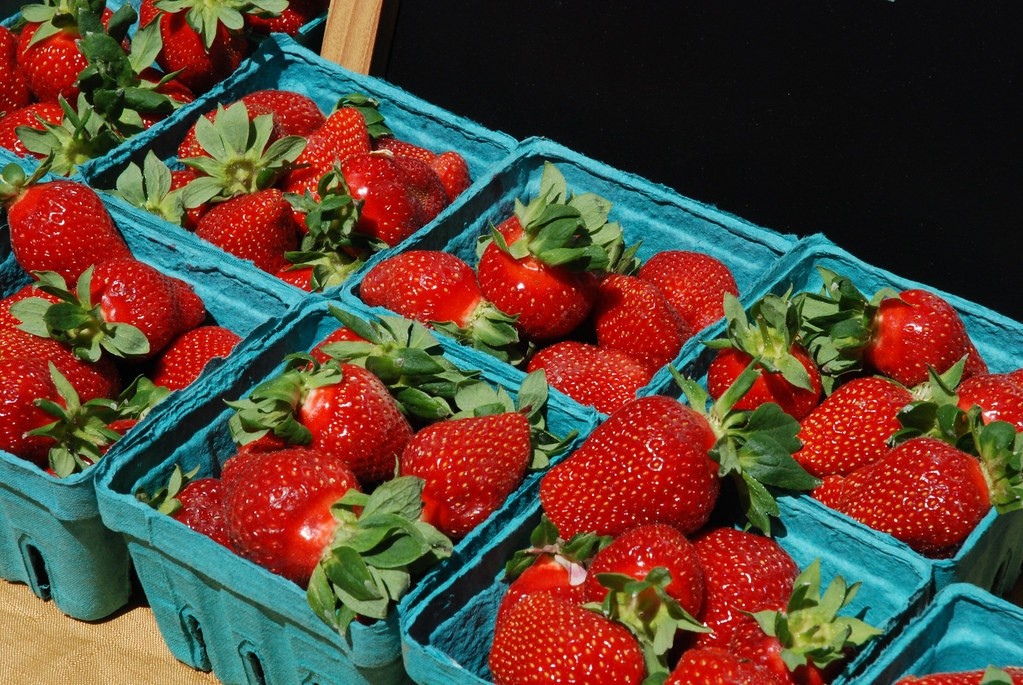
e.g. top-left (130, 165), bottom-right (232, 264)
top-left (0, 0), bottom-right (1023, 685)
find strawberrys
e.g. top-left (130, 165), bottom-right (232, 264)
top-left (0, 0), bottom-right (1023, 685)
